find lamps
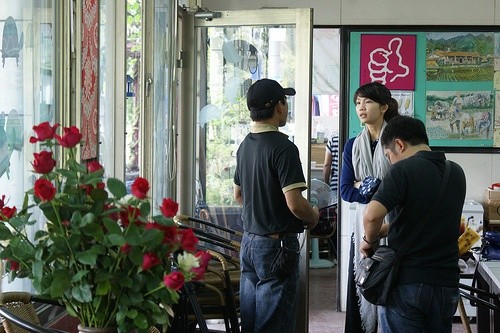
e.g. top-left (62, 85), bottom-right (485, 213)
top-left (126, 75), bottom-right (135, 96)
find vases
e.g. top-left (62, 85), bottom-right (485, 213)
top-left (77, 319), bottom-right (118, 333)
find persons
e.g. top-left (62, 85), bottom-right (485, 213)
top-left (234, 78), bottom-right (318, 333)
top-left (324, 135), bottom-right (338, 264)
top-left (360, 117), bottom-right (467, 333)
top-left (340, 83), bottom-right (400, 333)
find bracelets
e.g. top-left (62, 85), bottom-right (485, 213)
top-left (363, 235), bottom-right (379, 244)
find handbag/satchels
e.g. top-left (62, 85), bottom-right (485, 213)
top-left (355, 246), bottom-right (400, 306)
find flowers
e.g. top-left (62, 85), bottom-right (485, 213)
top-left (0, 120), bottom-right (209, 320)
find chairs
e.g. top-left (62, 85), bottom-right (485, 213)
top-left (0, 291), bottom-right (75, 333)
top-left (170, 214), bottom-right (277, 333)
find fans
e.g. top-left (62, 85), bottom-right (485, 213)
top-left (308, 179), bottom-right (333, 268)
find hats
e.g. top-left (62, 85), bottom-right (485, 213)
top-left (247, 79), bottom-right (296, 110)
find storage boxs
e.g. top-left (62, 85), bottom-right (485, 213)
top-left (485, 186), bottom-right (500, 224)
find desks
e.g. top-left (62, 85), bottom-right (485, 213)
top-left (473, 261), bottom-right (500, 333)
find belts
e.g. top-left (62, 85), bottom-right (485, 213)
top-left (268, 232), bottom-right (298, 238)
